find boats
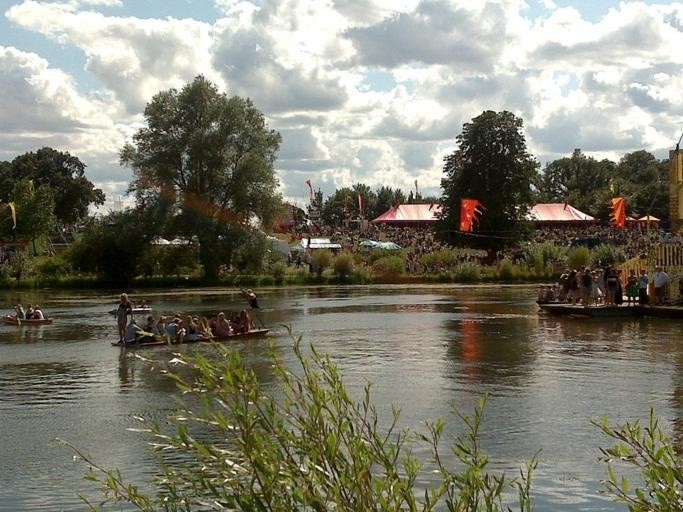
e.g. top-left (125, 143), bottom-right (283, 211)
top-left (111, 328), bottom-right (270, 347)
top-left (1, 315), bottom-right (54, 325)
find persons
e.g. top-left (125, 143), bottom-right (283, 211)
top-left (537, 263), bottom-right (669, 308)
top-left (531, 224), bottom-right (682, 260)
top-left (13, 303), bottom-right (44, 319)
top-left (452, 249), bottom-right (531, 269)
top-left (286, 216), bottom-right (450, 275)
top-left (114, 289), bottom-right (260, 344)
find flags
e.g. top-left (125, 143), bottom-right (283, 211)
top-left (306, 180), bottom-right (315, 204)
top-left (459, 199), bottom-right (485, 232)
top-left (611, 198), bottom-right (626, 227)
top-left (357, 193), bottom-right (363, 214)
